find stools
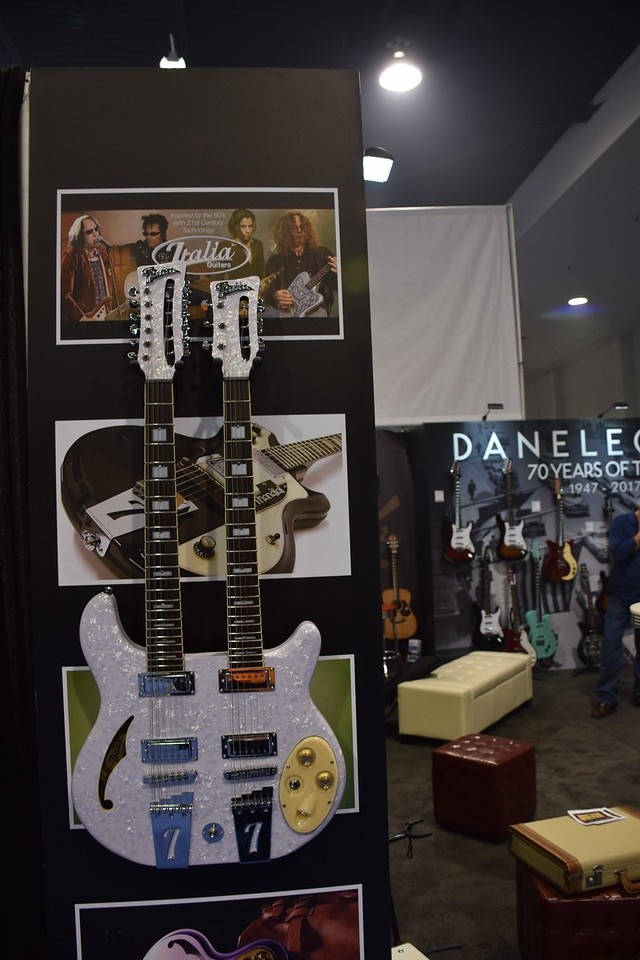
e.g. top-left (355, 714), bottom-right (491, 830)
top-left (432, 733), bottom-right (537, 843)
top-left (516, 859), bottom-right (640, 960)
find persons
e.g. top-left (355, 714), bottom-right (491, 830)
top-left (61, 208), bottom-right (337, 322)
top-left (591, 504), bottom-right (640, 718)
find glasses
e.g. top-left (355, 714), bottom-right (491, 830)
top-left (82, 226), bottom-right (99, 235)
top-left (143, 231), bottom-right (161, 236)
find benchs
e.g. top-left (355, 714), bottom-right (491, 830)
top-left (396, 651), bottom-right (534, 742)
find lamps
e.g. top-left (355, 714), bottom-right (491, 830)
top-left (380, 48), bottom-right (422, 92)
top-left (362, 146), bottom-right (393, 184)
top-left (158, 32), bottom-right (185, 68)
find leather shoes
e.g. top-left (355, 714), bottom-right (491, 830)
top-left (590, 702), bottom-right (617, 718)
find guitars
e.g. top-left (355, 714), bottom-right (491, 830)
top-left (57, 418), bottom-right (343, 579)
top-left (444, 459), bottom-right (579, 660)
top-left (71, 261), bottom-right (348, 870)
top-left (381, 534), bottom-right (419, 640)
top-left (576, 563), bottom-right (607, 665)
